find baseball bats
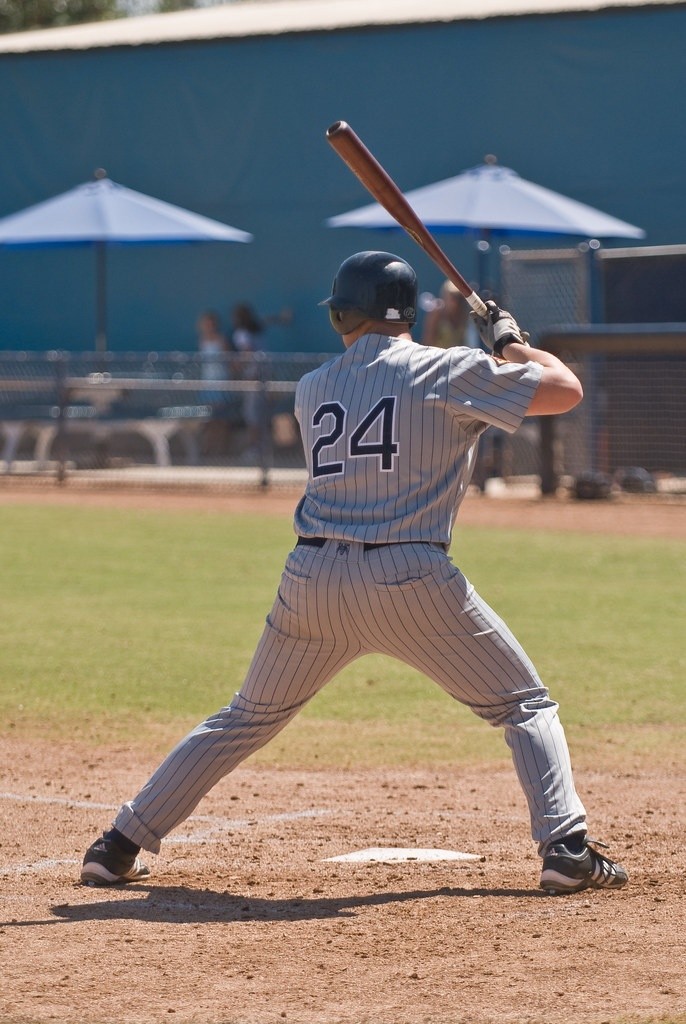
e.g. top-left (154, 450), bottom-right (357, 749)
top-left (326, 119), bottom-right (488, 321)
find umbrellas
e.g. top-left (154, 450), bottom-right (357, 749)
top-left (322, 153), bottom-right (645, 345)
top-left (0, 165), bottom-right (254, 349)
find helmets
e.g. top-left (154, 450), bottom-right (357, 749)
top-left (315, 250), bottom-right (418, 323)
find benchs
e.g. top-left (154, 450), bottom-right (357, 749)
top-left (0, 418), bottom-right (200, 476)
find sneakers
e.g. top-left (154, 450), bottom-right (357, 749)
top-left (80, 837), bottom-right (150, 885)
top-left (540, 836), bottom-right (628, 893)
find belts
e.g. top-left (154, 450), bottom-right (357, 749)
top-left (295, 535), bottom-right (428, 553)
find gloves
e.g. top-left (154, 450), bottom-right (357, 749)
top-left (469, 299), bottom-right (530, 363)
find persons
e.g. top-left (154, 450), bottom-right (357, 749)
top-left (190, 278), bottom-right (468, 457)
top-left (77, 250), bottom-right (631, 894)
